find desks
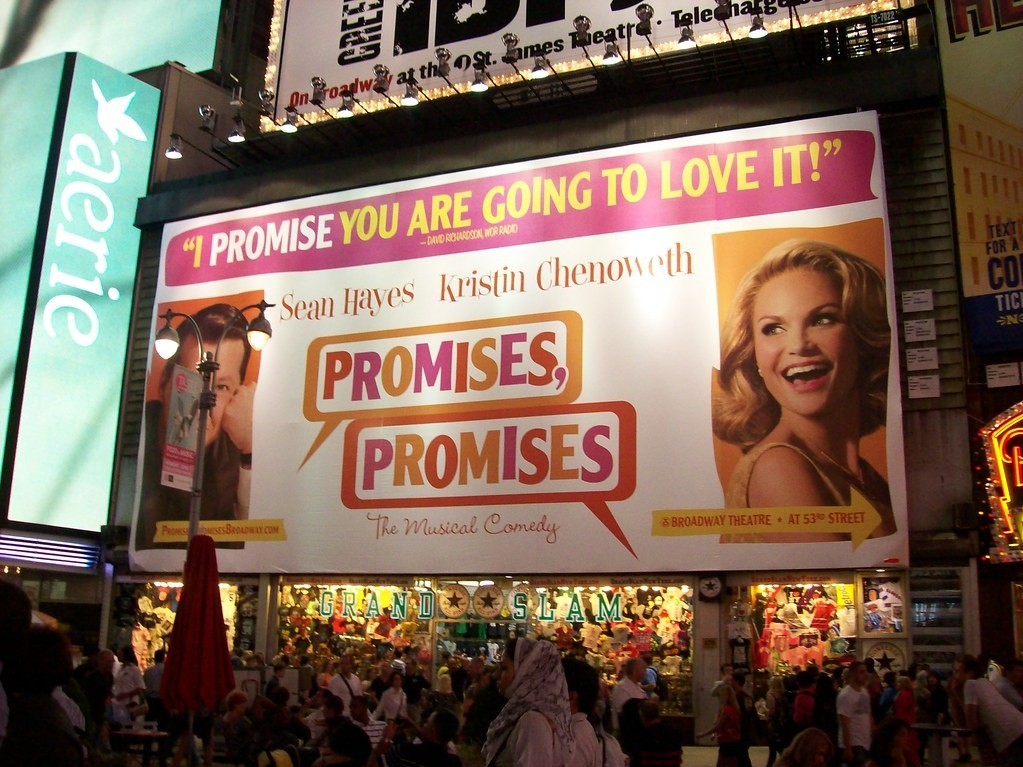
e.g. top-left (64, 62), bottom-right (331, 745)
top-left (910, 723), bottom-right (972, 767)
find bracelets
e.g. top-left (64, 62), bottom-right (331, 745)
top-left (240, 453), bottom-right (252, 470)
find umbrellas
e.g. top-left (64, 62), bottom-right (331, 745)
top-left (161, 536), bottom-right (238, 754)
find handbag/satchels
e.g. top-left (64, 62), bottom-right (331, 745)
top-left (125, 688), bottom-right (149, 716)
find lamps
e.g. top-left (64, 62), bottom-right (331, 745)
top-left (749, 17), bottom-right (769, 38)
top-left (401, 82), bottom-right (419, 106)
top-left (256, 89), bottom-right (275, 117)
top-left (470, 69), bottom-right (488, 92)
top-left (336, 95), bottom-right (354, 118)
top-left (501, 33), bottom-right (520, 64)
top-left (602, 44), bottom-right (620, 65)
top-left (434, 48), bottom-right (452, 78)
top-left (678, 28), bottom-right (697, 49)
top-left (309, 77), bottom-right (327, 105)
top-left (165, 137), bottom-right (183, 159)
top-left (281, 111), bottom-right (298, 133)
top-left (371, 64), bottom-right (390, 93)
top-left (227, 124), bottom-right (246, 142)
top-left (531, 58), bottom-right (548, 79)
top-left (573, 15), bottom-right (592, 46)
top-left (635, 4), bottom-right (654, 35)
top-left (198, 105), bottom-right (215, 133)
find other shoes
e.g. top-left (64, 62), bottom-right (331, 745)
top-left (955, 753), bottom-right (972, 763)
top-left (949, 740), bottom-right (958, 748)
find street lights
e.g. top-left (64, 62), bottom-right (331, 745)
top-left (152, 300), bottom-right (277, 549)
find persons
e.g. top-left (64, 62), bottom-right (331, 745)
top-left (712, 238), bottom-right (896, 543)
top-left (146, 302), bottom-right (257, 548)
top-left (0, 582), bottom-right (1023, 767)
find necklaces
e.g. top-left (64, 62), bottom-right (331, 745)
top-left (777, 413), bottom-right (878, 501)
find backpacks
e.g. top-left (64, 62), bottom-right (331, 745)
top-left (648, 667), bottom-right (671, 702)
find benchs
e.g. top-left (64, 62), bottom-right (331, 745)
top-left (309, 721), bottom-right (392, 767)
top-left (112, 722), bottom-right (169, 767)
